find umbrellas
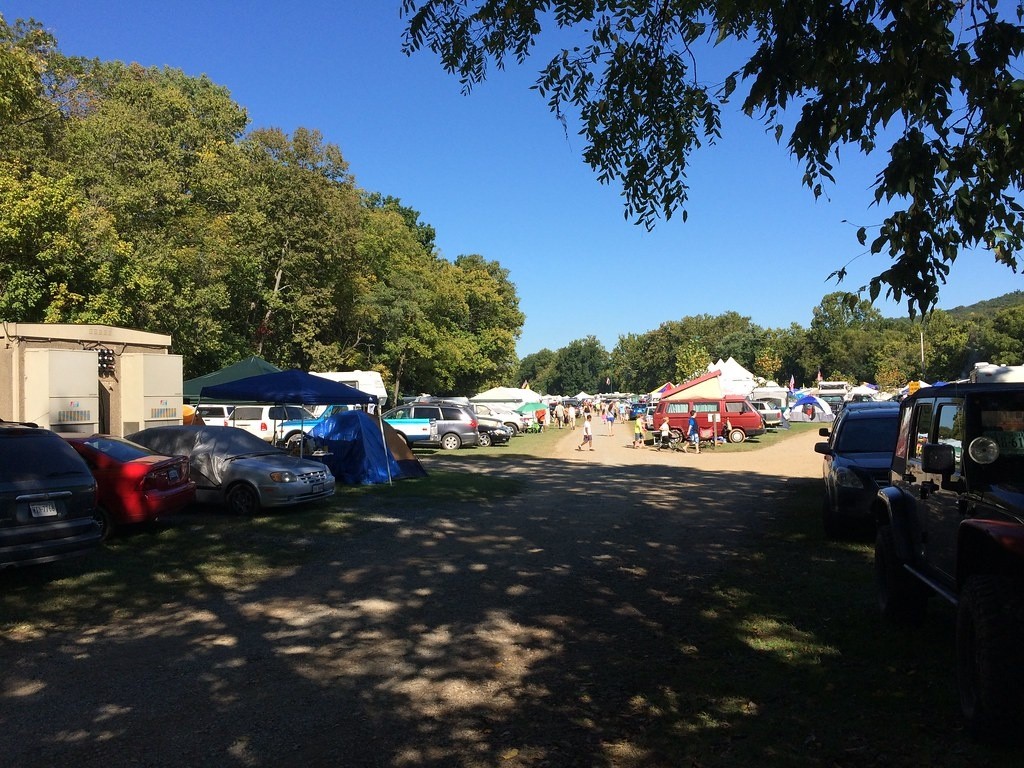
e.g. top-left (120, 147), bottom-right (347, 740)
top-left (515, 403), bottom-right (549, 433)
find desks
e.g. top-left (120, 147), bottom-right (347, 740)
top-left (297, 453), bottom-right (335, 475)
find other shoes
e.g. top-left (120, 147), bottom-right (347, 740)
top-left (578, 446), bottom-right (582, 450)
top-left (589, 449), bottom-right (595, 451)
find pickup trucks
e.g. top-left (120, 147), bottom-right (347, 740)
top-left (275, 405), bottom-right (438, 453)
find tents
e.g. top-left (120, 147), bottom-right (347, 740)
top-left (471, 379), bottom-right (677, 425)
top-left (705, 357), bottom-right (952, 423)
top-left (181, 355), bottom-right (283, 449)
top-left (289, 409), bottom-right (430, 486)
top-left (190, 368), bottom-right (393, 486)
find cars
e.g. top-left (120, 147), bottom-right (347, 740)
top-left (815, 401), bottom-right (903, 537)
top-left (123, 425), bottom-right (335, 515)
top-left (628, 401), bottom-right (659, 420)
top-left (750, 401), bottom-right (783, 425)
top-left (56, 432), bottom-right (197, 542)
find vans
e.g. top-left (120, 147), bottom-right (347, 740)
top-left (654, 395), bottom-right (766, 443)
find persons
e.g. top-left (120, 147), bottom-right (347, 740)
top-left (632, 414), bottom-right (648, 448)
top-left (535, 410), bottom-right (547, 433)
top-left (553, 400), bottom-right (677, 437)
top-left (656, 416), bottom-right (676, 452)
top-left (682, 410), bottom-right (702, 454)
top-left (576, 415), bottom-right (595, 451)
top-left (633, 413), bottom-right (644, 450)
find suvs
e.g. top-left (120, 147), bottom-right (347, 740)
top-left (875, 382), bottom-right (1024, 745)
top-left (0, 418), bottom-right (102, 570)
top-left (191, 404), bottom-right (354, 450)
top-left (378, 400), bottom-right (523, 450)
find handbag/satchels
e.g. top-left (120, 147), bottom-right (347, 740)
top-left (614, 413), bottom-right (616, 418)
